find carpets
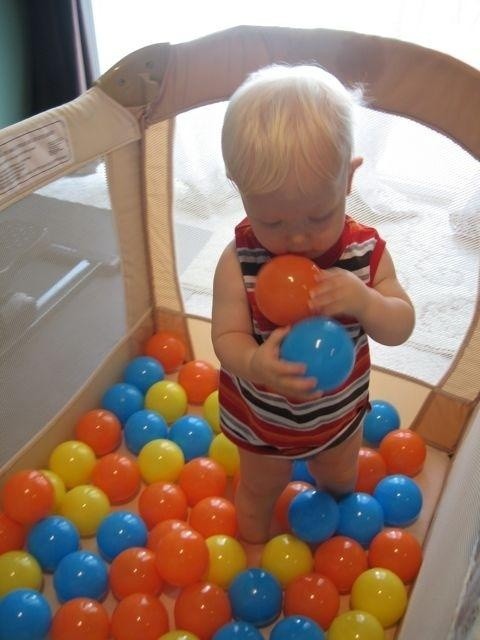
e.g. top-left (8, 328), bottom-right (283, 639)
top-left (178, 197), bottom-right (480, 357)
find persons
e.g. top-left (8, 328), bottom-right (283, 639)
top-left (210, 64), bottom-right (418, 574)
